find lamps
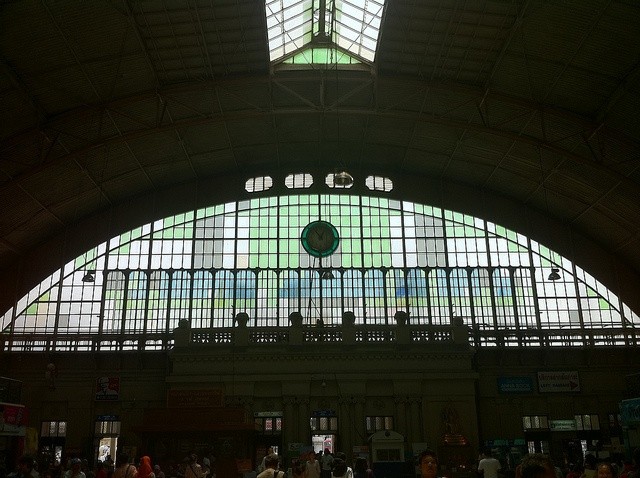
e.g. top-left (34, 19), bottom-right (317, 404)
top-left (548, 269), bottom-right (561, 280)
top-left (82, 269), bottom-right (97, 283)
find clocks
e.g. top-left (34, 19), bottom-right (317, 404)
top-left (301, 220), bottom-right (339, 257)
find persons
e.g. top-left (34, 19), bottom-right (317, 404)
top-left (629, 449), bottom-right (640, 478)
top-left (595, 463), bottom-right (615, 478)
top-left (256, 454), bottom-right (287, 478)
top-left (96, 463), bottom-right (110, 478)
top-left (185, 454), bottom-right (209, 478)
top-left (96, 377), bottom-right (117, 395)
top-left (478, 450), bottom-right (501, 477)
top-left (112, 456), bottom-right (138, 478)
top-left (261, 448), bottom-right (280, 471)
top-left (153, 465), bottom-right (165, 478)
top-left (583, 458), bottom-right (597, 478)
top-left (9, 458), bottom-right (39, 478)
top-left (566, 464), bottom-right (584, 478)
top-left (306, 451), bottom-right (321, 478)
top-left (64, 458), bottom-right (87, 478)
top-left (444, 412), bottom-right (464, 439)
top-left (135, 456), bottom-right (155, 478)
top-left (355, 457), bottom-right (374, 478)
top-left (419, 449), bottom-right (438, 478)
top-left (331, 452), bottom-right (353, 478)
top-left (105, 455), bottom-right (114, 466)
top-left (321, 448), bottom-right (335, 478)
top-left (516, 453), bottom-right (563, 478)
top-left (614, 453), bottom-right (636, 478)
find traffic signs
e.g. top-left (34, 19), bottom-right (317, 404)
top-left (537, 371), bottom-right (581, 391)
top-left (496, 376), bottom-right (532, 393)
